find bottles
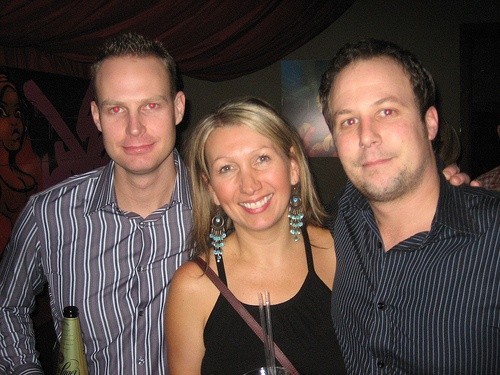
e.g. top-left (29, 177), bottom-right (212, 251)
top-left (58, 306), bottom-right (89, 375)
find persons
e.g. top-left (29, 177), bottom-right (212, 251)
top-left (318, 38), bottom-right (500, 375)
top-left (0, 30), bottom-right (199, 375)
top-left (163, 95), bottom-right (483, 375)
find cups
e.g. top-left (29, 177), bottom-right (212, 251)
top-left (244, 367), bottom-right (288, 375)
